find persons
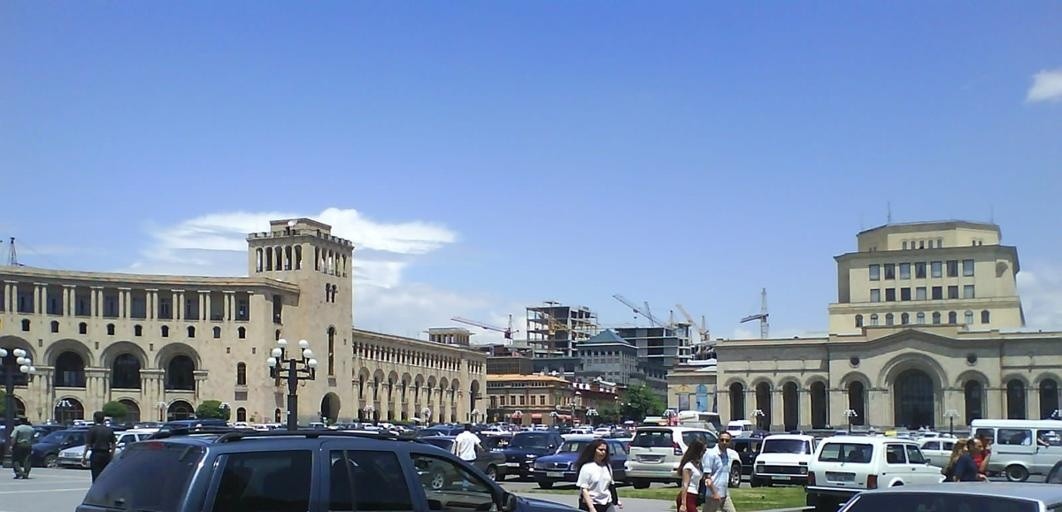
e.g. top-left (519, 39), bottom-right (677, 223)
top-left (81, 410), bottom-right (117, 483)
top-left (450, 424), bottom-right (485, 491)
top-left (10, 414), bottom-right (33, 477)
top-left (575, 440), bottom-right (624, 511)
top-left (701, 431), bottom-right (737, 511)
top-left (676, 439), bottom-right (713, 511)
top-left (940, 430), bottom-right (1050, 481)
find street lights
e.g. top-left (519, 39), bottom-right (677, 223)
top-left (840, 409), bottom-right (858, 434)
top-left (942, 408), bottom-right (960, 438)
top-left (0, 348), bottom-right (36, 468)
top-left (363, 405), bottom-right (375, 422)
top-left (219, 402), bottom-right (232, 421)
top-left (152, 401), bottom-right (169, 422)
top-left (749, 409), bottom-right (765, 434)
top-left (471, 402), bottom-right (677, 426)
top-left (1051, 409), bottom-right (1062, 420)
top-left (57, 400), bottom-right (72, 422)
top-left (267, 338), bottom-right (317, 429)
top-left (420, 407), bottom-right (431, 426)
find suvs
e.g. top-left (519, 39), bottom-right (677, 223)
top-left (623, 426), bottom-right (742, 489)
top-left (76, 425), bottom-right (588, 512)
top-left (803, 434), bottom-right (947, 512)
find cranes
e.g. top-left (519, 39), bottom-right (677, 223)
top-left (450, 310), bottom-right (520, 342)
top-left (675, 304), bottom-right (710, 340)
top-left (740, 287), bottom-right (773, 338)
top-left (611, 292), bottom-right (677, 336)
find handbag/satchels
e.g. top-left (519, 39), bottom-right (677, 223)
top-left (609, 484), bottom-right (618, 505)
top-left (697, 479), bottom-right (706, 506)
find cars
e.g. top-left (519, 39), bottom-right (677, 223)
top-left (1045, 459), bottom-right (1062, 484)
top-left (534, 440), bottom-right (633, 489)
top-left (750, 434), bottom-right (817, 487)
top-left (837, 481), bottom-right (1062, 512)
